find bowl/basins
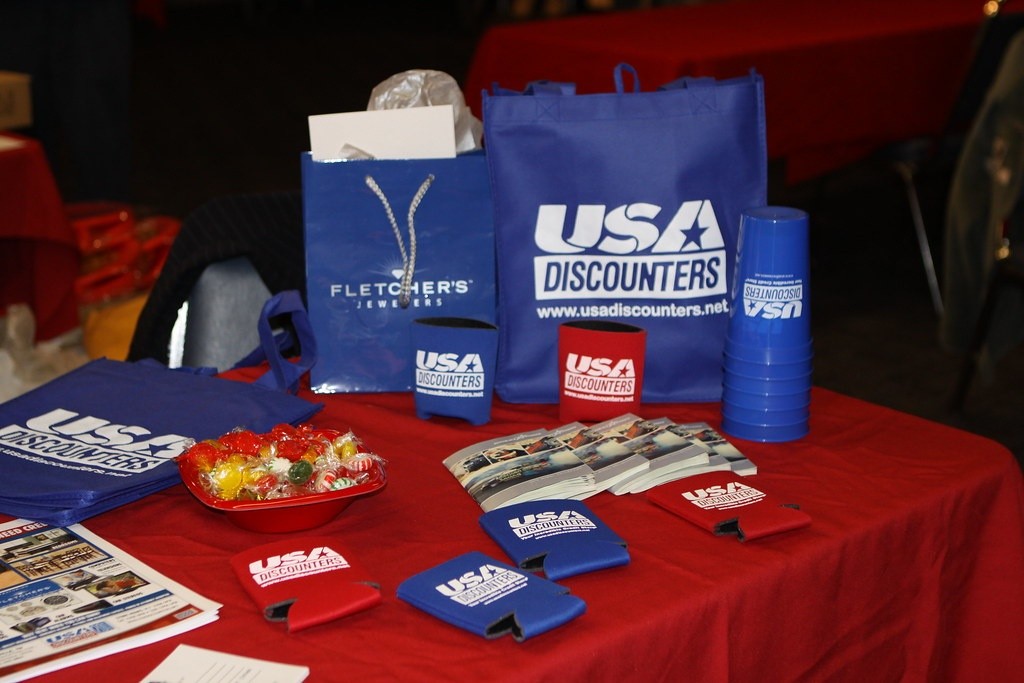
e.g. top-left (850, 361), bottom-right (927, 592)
top-left (179, 429), bottom-right (386, 533)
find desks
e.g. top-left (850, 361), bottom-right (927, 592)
top-left (0, 355), bottom-right (1024, 683)
top-left (467, 1), bottom-right (1023, 302)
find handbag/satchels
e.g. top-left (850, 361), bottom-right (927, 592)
top-left (480, 63), bottom-right (767, 404)
top-left (301, 149), bottom-right (497, 396)
top-left (0, 291), bottom-right (325, 528)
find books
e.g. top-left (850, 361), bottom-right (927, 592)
top-left (631, 416), bottom-right (731, 495)
top-left (0, 520), bottom-right (224, 683)
top-left (588, 411), bottom-right (710, 496)
top-left (443, 428), bottom-right (597, 513)
top-left (549, 422), bottom-right (650, 501)
top-left (680, 422), bottom-right (757, 477)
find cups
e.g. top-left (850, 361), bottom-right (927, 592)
top-left (720, 206), bottom-right (815, 443)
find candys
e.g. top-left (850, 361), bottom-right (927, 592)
top-left (180, 423), bottom-right (376, 500)
